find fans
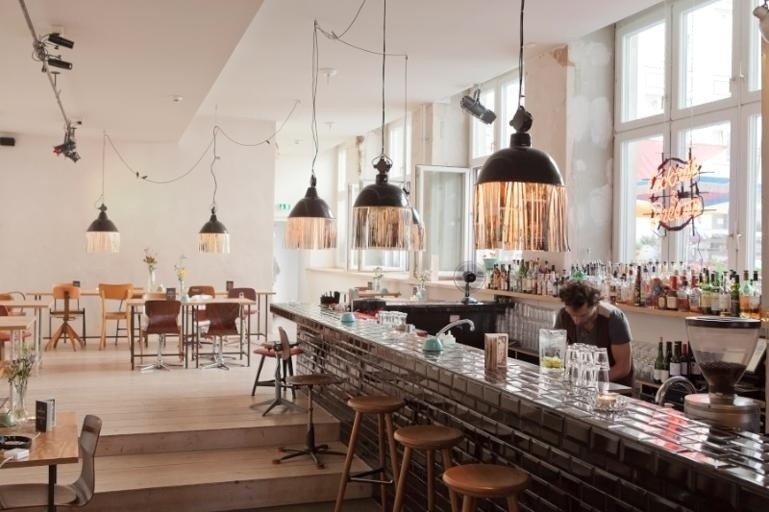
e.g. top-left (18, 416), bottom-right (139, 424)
top-left (454, 259), bottom-right (485, 303)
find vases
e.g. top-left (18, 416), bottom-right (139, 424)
top-left (179, 280), bottom-right (185, 295)
top-left (418, 287), bottom-right (425, 301)
top-left (148, 270), bottom-right (156, 291)
top-left (375, 278), bottom-right (380, 291)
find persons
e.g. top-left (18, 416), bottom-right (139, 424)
top-left (554, 280), bottom-right (634, 396)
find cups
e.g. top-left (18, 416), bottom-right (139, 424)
top-left (538, 329), bottom-right (567, 373)
top-left (566, 342), bottom-right (609, 395)
top-left (377, 310), bottom-right (407, 331)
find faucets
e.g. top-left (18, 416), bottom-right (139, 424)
top-left (655, 373), bottom-right (698, 404)
top-left (436, 319), bottom-right (476, 337)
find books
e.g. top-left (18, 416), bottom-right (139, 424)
top-left (35, 399), bottom-right (56, 432)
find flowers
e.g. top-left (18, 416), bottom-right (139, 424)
top-left (412, 270), bottom-right (431, 287)
top-left (137, 245), bottom-right (160, 275)
top-left (372, 267), bottom-right (383, 278)
top-left (173, 252), bottom-right (191, 283)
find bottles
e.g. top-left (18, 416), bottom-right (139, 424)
top-left (489, 257), bottom-right (761, 319)
top-left (653, 340), bottom-right (696, 383)
top-left (239, 291), bottom-right (245, 301)
top-left (368, 281), bottom-right (372, 290)
top-left (412, 285), bottom-right (418, 295)
top-left (436, 329), bottom-right (458, 346)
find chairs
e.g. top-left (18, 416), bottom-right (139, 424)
top-left (0, 282), bottom-right (276, 416)
top-left (0, 415), bottom-right (102, 512)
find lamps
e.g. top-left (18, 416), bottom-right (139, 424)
top-left (472, 0), bottom-right (571, 252)
top-left (287, 0), bottom-right (426, 250)
top-left (199, 127), bottom-right (230, 253)
top-left (85, 131), bottom-right (121, 255)
